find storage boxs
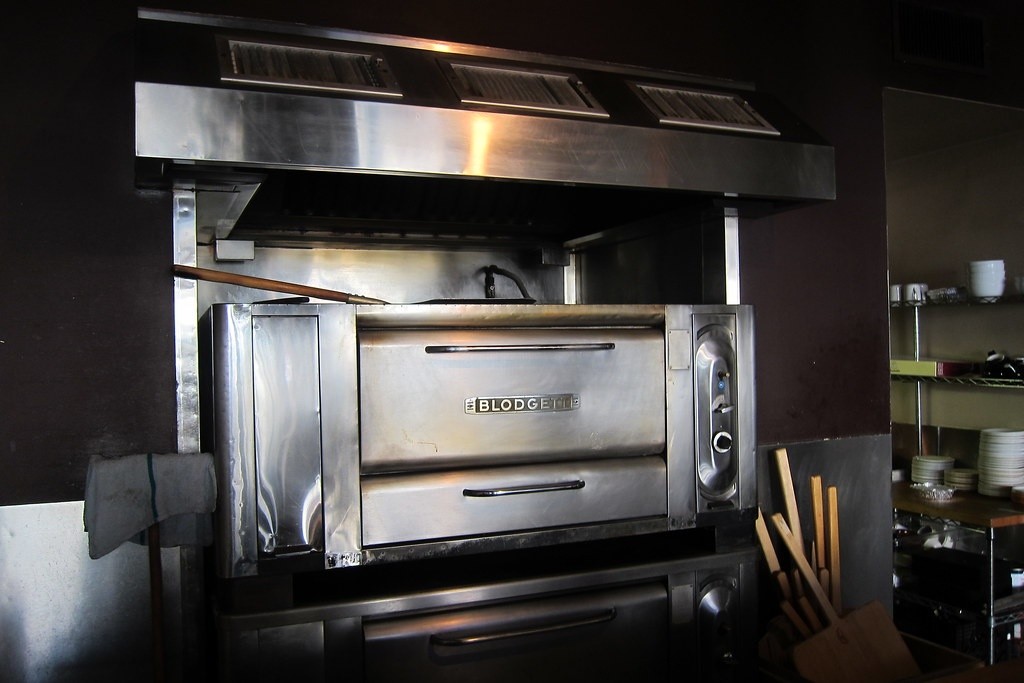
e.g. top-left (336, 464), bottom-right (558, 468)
top-left (889, 359), bottom-right (971, 378)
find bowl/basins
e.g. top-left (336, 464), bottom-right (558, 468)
top-left (927, 287), bottom-right (959, 306)
top-left (967, 258), bottom-right (1006, 304)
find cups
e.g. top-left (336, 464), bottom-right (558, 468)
top-left (890, 283), bottom-right (927, 307)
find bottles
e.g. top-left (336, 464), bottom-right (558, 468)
top-left (986, 350), bottom-right (1000, 363)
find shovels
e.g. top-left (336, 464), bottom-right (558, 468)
top-left (172, 261), bottom-right (538, 305)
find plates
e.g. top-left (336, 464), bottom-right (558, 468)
top-left (910, 427), bottom-right (1024, 496)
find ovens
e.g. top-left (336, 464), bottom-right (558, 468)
top-left (130, 7), bottom-right (894, 683)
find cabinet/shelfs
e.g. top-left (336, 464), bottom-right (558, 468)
top-left (891, 482), bottom-right (1024, 665)
top-left (891, 295), bottom-right (1024, 457)
